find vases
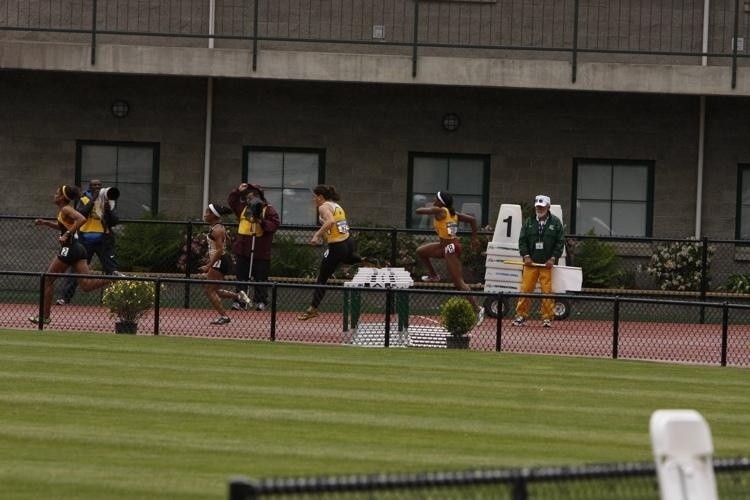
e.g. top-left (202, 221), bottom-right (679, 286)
top-left (115, 321), bottom-right (138, 335)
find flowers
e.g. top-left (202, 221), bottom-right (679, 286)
top-left (102, 275), bottom-right (168, 322)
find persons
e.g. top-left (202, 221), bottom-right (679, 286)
top-left (28, 184), bottom-right (125, 324)
top-left (228, 183), bottom-right (280, 312)
top-left (296, 184), bottom-right (376, 320)
top-left (415, 191), bottom-right (486, 326)
top-left (197, 203), bottom-right (253, 324)
top-left (512, 195), bottom-right (565, 328)
top-left (57, 180), bottom-right (120, 306)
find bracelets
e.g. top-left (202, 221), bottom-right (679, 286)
top-left (523, 254), bottom-right (530, 258)
top-left (65, 230), bottom-right (71, 236)
top-left (550, 256), bottom-right (556, 261)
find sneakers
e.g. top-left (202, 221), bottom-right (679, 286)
top-left (238, 289), bottom-right (254, 308)
top-left (511, 315), bottom-right (528, 327)
top-left (56, 298), bottom-right (69, 306)
top-left (255, 302), bottom-right (265, 311)
top-left (28, 314), bottom-right (52, 324)
top-left (421, 274), bottom-right (442, 282)
top-left (542, 318), bottom-right (552, 328)
top-left (476, 305), bottom-right (486, 327)
top-left (112, 270), bottom-right (127, 278)
top-left (231, 302), bottom-right (242, 310)
top-left (296, 308), bottom-right (321, 320)
top-left (210, 315), bottom-right (232, 325)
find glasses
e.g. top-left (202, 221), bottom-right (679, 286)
top-left (534, 198), bottom-right (543, 203)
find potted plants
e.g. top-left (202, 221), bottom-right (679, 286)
top-left (438, 295), bottom-right (477, 349)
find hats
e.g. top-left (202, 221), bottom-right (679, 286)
top-left (534, 195), bottom-right (551, 208)
top-left (247, 181), bottom-right (265, 196)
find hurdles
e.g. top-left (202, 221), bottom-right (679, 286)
top-left (342, 267), bottom-right (472, 350)
